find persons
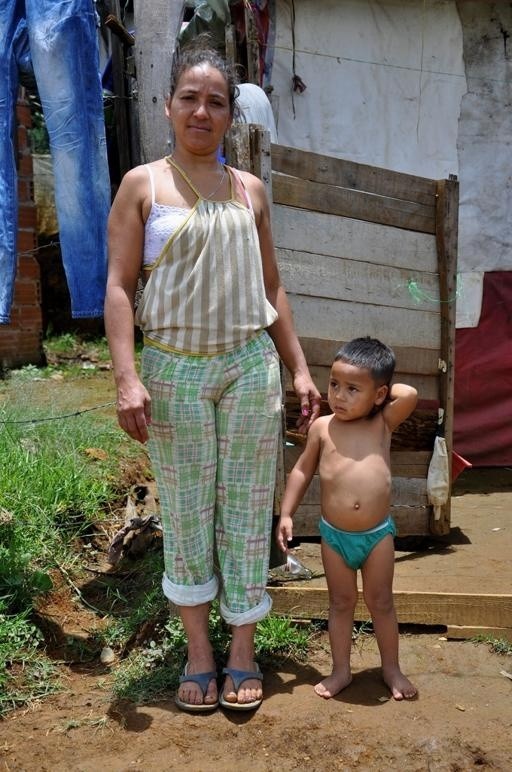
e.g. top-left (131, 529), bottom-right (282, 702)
top-left (104, 48), bottom-right (320, 713)
top-left (274, 336), bottom-right (420, 701)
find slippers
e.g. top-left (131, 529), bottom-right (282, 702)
top-left (219, 658), bottom-right (265, 712)
top-left (175, 658), bottom-right (220, 713)
